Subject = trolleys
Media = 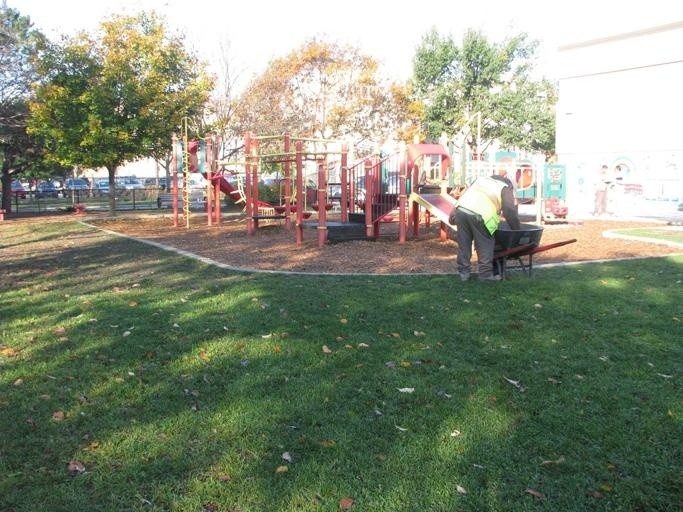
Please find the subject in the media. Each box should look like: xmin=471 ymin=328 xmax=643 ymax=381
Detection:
xmin=493 ymin=220 xmax=579 ymax=282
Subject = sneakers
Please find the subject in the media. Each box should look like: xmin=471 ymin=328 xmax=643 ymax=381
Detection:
xmin=479 ymin=274 xmax=502 ymax=282
xmin=460 ymin=271 xmax=470 ymax=281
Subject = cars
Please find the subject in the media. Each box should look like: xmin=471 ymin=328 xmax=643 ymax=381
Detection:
xmin=0 ymin=174 xmax=201 ymax=208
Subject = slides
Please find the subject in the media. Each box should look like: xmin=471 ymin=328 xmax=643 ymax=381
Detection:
xmin=201 ymin=172 xmax=272 ymax=207
xmin=410 ymin=191 xmax=458 ymax=231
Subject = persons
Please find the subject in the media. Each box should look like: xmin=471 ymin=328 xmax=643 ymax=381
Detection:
xmin=594 ymin=164 xmax=615 ymax=216
xmin=453 ymin=175 xmax=522 ymax=282
xmin=615 ymin=166 xmax=624 ymax=180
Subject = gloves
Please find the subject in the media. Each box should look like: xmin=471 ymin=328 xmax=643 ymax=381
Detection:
xmin=449 ymin=209 xmax=457 ymax=225
